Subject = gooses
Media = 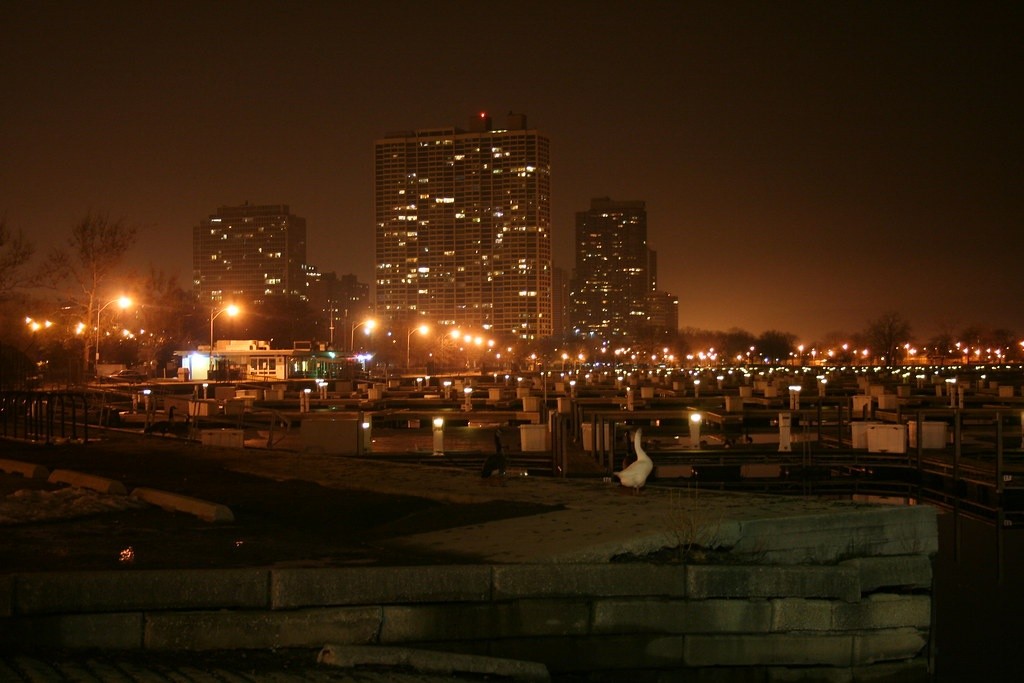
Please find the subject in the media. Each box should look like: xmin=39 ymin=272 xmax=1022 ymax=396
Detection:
xmin=612 ymin=427 xmax=656 ymax=497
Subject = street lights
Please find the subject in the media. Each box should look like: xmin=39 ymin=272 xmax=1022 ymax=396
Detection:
xmin=440 ymin=330 xmax=461 ymax=364
xmin=209 ymin=303 xmax=238 ymax=357
xmin=405 ymin=326 xmax=429 ymax=371
xmin=349 ymin=319 xmax=377 ymax=354
xmin=94 ymin=296 xmax=132 ymax=371
